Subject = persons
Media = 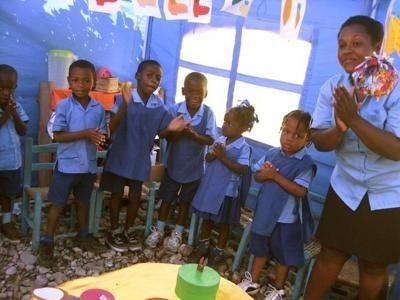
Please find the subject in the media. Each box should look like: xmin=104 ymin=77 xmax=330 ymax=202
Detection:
xmin=190 ymin=99 xmax=258 ymax=264
xmin=144 ymin=70 xmax=217 ymax=254
xmin=235 ymin=110 xmax=317 ymax=300
xmin=0 ymin=63 xmax=29 ymax=244
xmin=39 ymin=59 xmax=109 ymax=260
xmin=98 ymin=60 xmax=187 ymax=253
xmin=302 ymin=14 xmax=400 ymax=300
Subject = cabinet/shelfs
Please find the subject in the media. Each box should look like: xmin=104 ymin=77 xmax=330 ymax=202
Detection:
xmin=38 ymin=81 xmax=165 ymax=213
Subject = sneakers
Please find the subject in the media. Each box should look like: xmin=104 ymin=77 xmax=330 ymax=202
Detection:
xmin=75 ymin=232 xmax=107 ymax=254
xmin=2 ymin=221 xmax=20 ymax=240
xmin=106 ymin=228 xmax=129 ymax=253
xmin=236 ymin=270 xmax=261 ymax=296
xmin=122 ymin=227 xmax=142 ymax=251
xmin=264 ymin=283 xmax=287 ymax=300
xmin=165 ymin=229 xmax=183 ymax=252
xmin=37 ymin=235 xmax=55 ymax=266
xmin=144 ymin=226 xmax=165 ymax=249
xmin=187 ymin=246 xmax=211 ymax=262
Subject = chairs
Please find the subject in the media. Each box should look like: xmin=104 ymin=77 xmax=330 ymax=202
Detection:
xmin=231 ymin=215 xmax=322 ymax=300
xmin=302 ymin=255 xmax=400 ymax=300
xmin=151 ymin=181 xmax=199 ymax=245
xmin=195 ymin=186 xmax=260 ymax=272
xmin=21 ymin=137 xmax=96 ymax=249
xmin=94 ymin=151 xmax=153 ymax=236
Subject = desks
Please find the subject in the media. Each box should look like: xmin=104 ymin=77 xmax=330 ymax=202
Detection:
xmin=31 ymin=263 xmax=255 ymax=300
